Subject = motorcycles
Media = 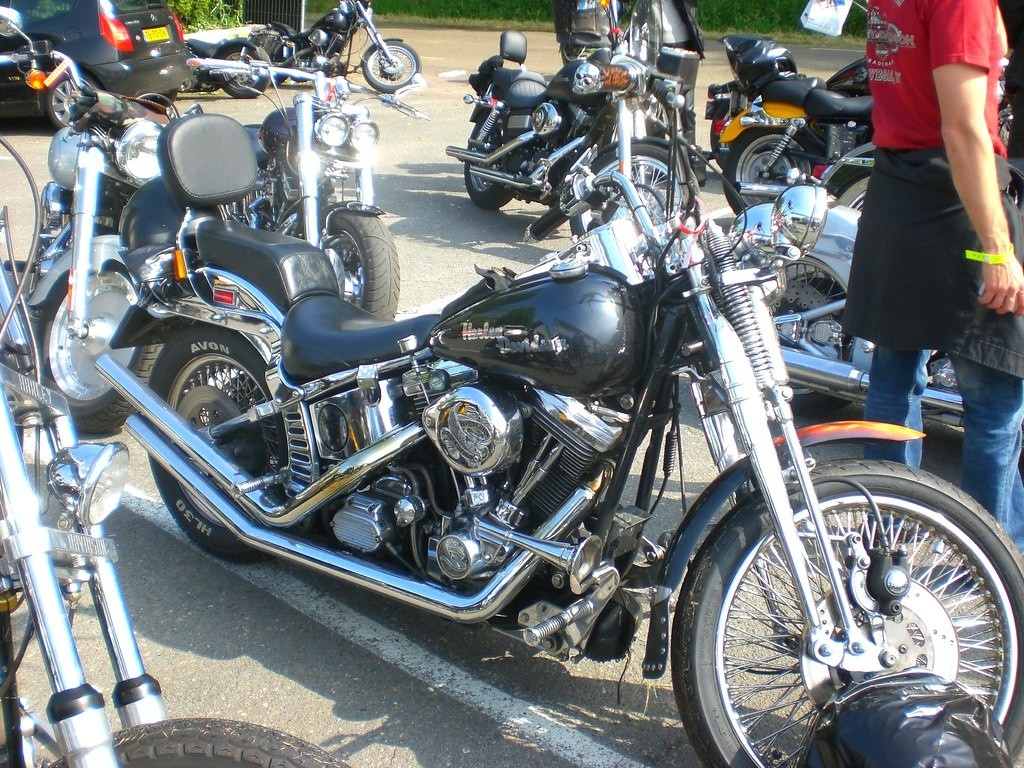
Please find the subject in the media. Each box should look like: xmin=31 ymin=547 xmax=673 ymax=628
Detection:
xmin=440 ymin=0 xmax=1024 ymax=468
xmin=1 ymin=134 xmax=351 ymax=768
xmin=1 ymin=4 xmax=207 ymax=438
xmin=182 ymin=49 xmax=427 ymax=321
xmin=181 ymin=31 xmax=274 ymax=99
xmin=249 ymin=0 xmax=423 ymax=94
xmin=111 ymin=36 xmax=1024 ymax=768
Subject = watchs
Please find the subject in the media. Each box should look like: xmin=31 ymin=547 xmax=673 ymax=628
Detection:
xmin=966 ymin=244 xmax=1015 ymax=264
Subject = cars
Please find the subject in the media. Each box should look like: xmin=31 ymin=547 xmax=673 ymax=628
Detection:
xmin=2 ymin=0 xmax=195 ymax=131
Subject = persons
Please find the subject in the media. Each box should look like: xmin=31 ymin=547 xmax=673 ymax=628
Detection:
xmin=840 ymin=0 xmax=1024 ymax=548
xmin=633 ymin=0 xmax=707 ymax=190
xmin=554 ymin=0 xmax=624 ymax=67
